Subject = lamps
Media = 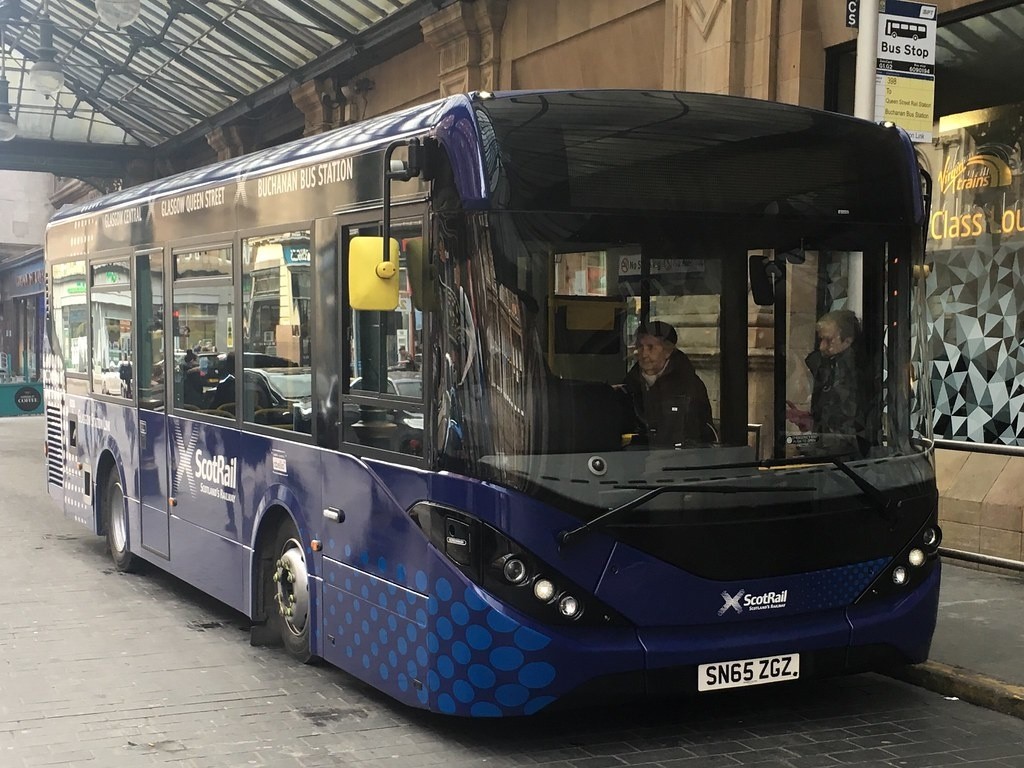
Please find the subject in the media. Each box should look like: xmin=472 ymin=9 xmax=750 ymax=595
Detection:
xmin=0 ymin=0 xmax=141 ymax=140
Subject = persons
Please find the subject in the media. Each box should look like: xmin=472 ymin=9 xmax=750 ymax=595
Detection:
xmin=179 ymin=349 xmax=203 ymax=410
xmin=210 ymin=351 xmax=246 ymax=533
xmin=613 ymin=321 xmax=717 ymax=451
xmin=786 ymin=309 xmax=884 ymax=458
xmin=397 ymin=346 xmax=417 ymax=371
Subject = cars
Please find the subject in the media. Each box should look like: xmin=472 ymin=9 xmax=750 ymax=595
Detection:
xmin=350 ymin=370 xmax=424 ymax=431
xmin=102 ymin=362 xmax=131 ymax=396
xmin=176 ymin=351 xmax=220 ymax=386
xmin=244 ymin=367 xmax=311 ymax=422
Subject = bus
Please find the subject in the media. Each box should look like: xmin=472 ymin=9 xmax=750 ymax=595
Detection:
xmin=43 ymin=87 xmax=943 ymax=724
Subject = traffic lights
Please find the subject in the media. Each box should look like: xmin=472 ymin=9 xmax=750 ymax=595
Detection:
xmin=155 ymin=308 xmax=163 ymax=327
xmin=170 ymin=309 xmax=182 ymax=338
